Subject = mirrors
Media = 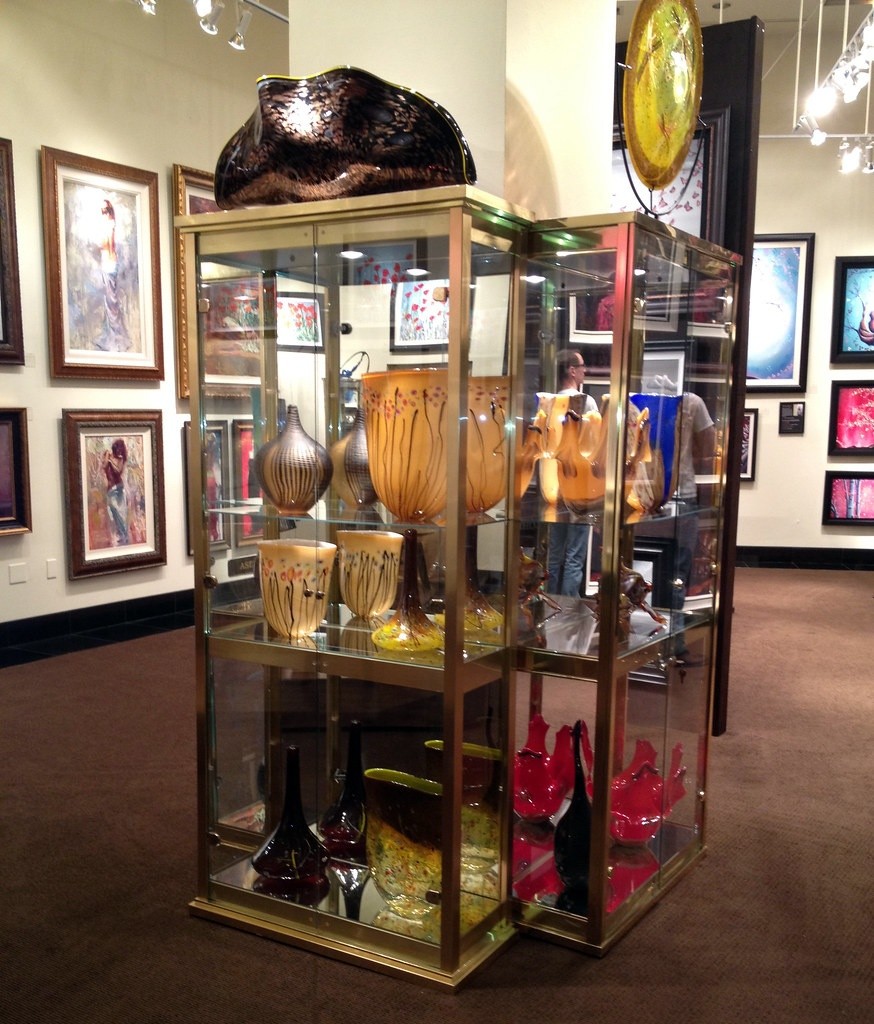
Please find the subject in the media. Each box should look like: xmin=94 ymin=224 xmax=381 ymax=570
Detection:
xmin=265 ymin=253 xmax=508 ymax=904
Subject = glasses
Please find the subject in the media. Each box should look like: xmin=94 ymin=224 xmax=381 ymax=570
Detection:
xmin=573 ymin=364 xmax=586 ymax=368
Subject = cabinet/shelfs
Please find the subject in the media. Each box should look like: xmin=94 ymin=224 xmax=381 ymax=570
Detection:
xmin=175 ymin=178 xmax=735 ymax=985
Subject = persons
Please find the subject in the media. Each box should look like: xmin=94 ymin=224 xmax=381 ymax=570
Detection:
xmin=532 ymin=348 xmax=605 ymax=601
xmin=634 ymin=355 xmax=718 ymax=665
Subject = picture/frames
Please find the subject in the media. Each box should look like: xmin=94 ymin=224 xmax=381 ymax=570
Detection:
xmin=276 ymin=289 xmax=327 ymax=354
xmin=0 ymin=406 xmax=34 ymax=537
xmin=829 ymin=255 xmax=874 ymax=365
xmin=820 ymin=470 xmax=873 ymax=525
xmin=737 ymin=407 xmax=758 ymax=482
xmin=41 ymin=142 xmax=166 ymax=383
xmin=61 ymin=406 xmax=168 ymax=581
xmin=741 ymin=231 xmax=814 ymax=392
xmin=171 ymin=161 xmax=281 ymax=399
xmin=0 ymin=136 xmax=26 ymax=367
xmin=826 ymin=380 xmax=874 ymax=456
xmin=778 ymin=401 xmax=805 ymax=434
xmin=184 ymin=417 xmax=272 ymax=555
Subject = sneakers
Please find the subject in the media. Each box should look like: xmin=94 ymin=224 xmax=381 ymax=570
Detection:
xmin=676 ymin=650 xmax=707 ymax=667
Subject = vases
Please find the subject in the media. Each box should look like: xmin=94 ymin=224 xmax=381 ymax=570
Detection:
xmin=333 ymin=530 xmax=406 ymax=622
xmin=456 ymin=373 xmax=511 ymax=523
xmin=251 ymin=743 xmax=330 ymax=882
xmin=330 ymin=394 xmax=380 ymax=513
xmin=319 ymin=718 xmax=369 ymax=855
xmin=256 ymin=539 xmax=338 ymax=636
xmin=361 ymin=369 xmax=456 ymax=521
xmin=246 ymin=400 xmax=332 ymax=514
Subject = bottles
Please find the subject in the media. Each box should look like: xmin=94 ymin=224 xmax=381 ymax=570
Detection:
xmin=324 ymin=848 xmax=371 ymax=922
xmin=251 ymin=876 xmax=330 ymax=910
xmin=250 ymin=745 xmax=330 ymax=879
xmin=318 ymin=719 xmax=370 ymax=844
xmin=372 ymin=529 xmax=445 ymax=653
xmin=252 ymin=405 xmax=334 ymax=515
xmin=330 ymin=409 xmax=378 ymax=508
xmin=434 ymin=525 xmax=503 ymax=645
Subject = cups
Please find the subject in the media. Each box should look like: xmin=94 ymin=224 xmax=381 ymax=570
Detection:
xmin=256 ymin=539 xmax=338 ymax=638
xmin=336 ymin=529 xmax=405 ymax=618
xmin=600 ymin=392 xmax=685 ymax=514
xmin=363 ymin=368 xmax=451 ymax=521
xmin=465 ymin=375 xmax=509 ymax=514
